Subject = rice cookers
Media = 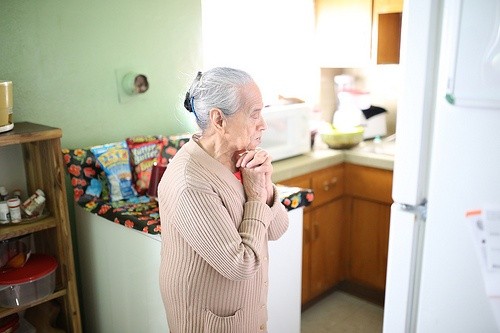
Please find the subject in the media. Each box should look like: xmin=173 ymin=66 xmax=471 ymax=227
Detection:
xmin=0 ymin=81 xmax=14 ymax=133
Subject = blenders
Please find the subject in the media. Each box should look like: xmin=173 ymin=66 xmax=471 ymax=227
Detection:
xmin=333 ymin=73 xmax=370 ymax=129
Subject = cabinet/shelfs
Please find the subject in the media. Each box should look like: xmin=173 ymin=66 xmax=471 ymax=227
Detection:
xmin=273 ymin=164 xmax=393 ymax=311
xmin=311 ymin=0 xmax=404 ymax=71
xmin=0 ymin=121 xmax=83 ymax=333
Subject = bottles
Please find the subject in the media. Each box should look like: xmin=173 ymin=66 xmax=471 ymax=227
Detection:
xmin=0 ymin=185 xmax=45 ymax=223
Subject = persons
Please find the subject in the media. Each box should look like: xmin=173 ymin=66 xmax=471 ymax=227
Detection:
xmin=159 ymin=67 xmax=289 ymax=333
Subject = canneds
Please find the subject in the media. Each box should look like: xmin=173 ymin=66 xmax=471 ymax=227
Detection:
xmin=6 ymin=189 xmax=46 ymax=223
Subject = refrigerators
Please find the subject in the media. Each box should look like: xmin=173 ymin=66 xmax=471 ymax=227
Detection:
xmin=381 ymin=0 xmax=500 ymax=333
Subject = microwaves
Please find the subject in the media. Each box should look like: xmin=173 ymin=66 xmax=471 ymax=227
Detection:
xmin=256 ymin=101 xmax=313 ymax=163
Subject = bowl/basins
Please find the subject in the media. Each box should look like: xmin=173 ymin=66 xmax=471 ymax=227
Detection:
xmin=319 ymin=126 xmax=364 ymax=149
xmin=0 ymin=253 xmax=59 ymax=309
